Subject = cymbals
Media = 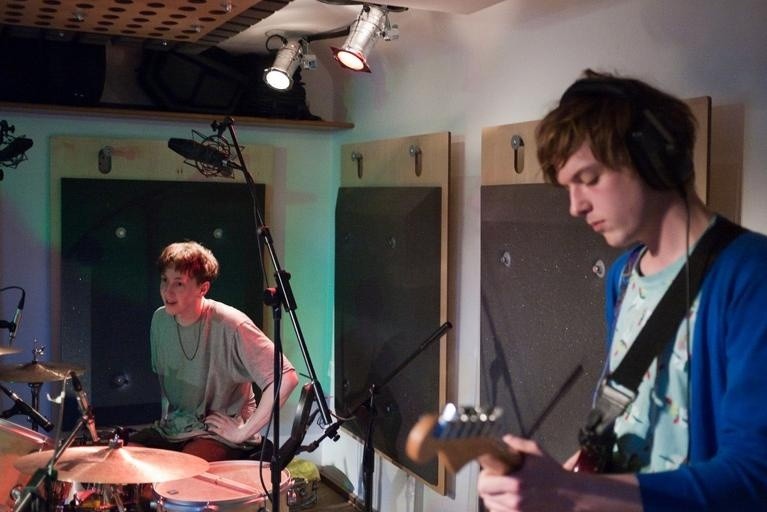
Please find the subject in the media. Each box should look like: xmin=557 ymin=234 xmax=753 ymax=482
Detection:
xmin=14 ymin=446 xmax=209 ymax=485
xmin=0 ymin=363 xmax=83 ymax=382
xmin=0 ymin=347 xmax=23 ymax=355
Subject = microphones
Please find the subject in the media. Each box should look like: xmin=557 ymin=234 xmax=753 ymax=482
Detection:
xmin=7 ymin=291 xmax=25 ymax=346
xmin=168 ymin=138 xmax=239 ymax=173
xmin=0 ymin=136 xmax=33 ymax=163
xmin=70 ymin=369 xmax=98 ymax=445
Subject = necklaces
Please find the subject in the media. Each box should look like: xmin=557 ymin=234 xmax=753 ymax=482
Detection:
xmin=176 ymin=300 xmax=206 ymax=361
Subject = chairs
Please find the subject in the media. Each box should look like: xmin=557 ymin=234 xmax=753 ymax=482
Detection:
xmin=233 ymin=381 xmax=272 ymax=460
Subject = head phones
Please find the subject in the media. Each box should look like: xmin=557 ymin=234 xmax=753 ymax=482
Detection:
xmin=565 ymin=75 xmax=693 ymax=189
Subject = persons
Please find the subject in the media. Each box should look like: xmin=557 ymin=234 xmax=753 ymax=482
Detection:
xmin=475 ymin=70 xmax=767 ymax=512
xmin=123 ymin=240 xmax=299 ymax=510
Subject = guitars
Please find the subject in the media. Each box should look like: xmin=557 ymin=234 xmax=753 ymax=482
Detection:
xmin=407 ymin=404 xmax=644 ymax=476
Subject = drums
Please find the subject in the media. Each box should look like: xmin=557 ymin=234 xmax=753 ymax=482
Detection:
xmin=148 ymin=460 xmax=290 ymax=512
xmin=0 ymin=417 xmax=58 ymax=511
xmin=281 ymin=459 xmax=320 ymax=510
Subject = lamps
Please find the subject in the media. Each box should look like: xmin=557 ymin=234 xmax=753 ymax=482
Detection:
xmin=262 ymin=3 xmax=399 ymax=92
